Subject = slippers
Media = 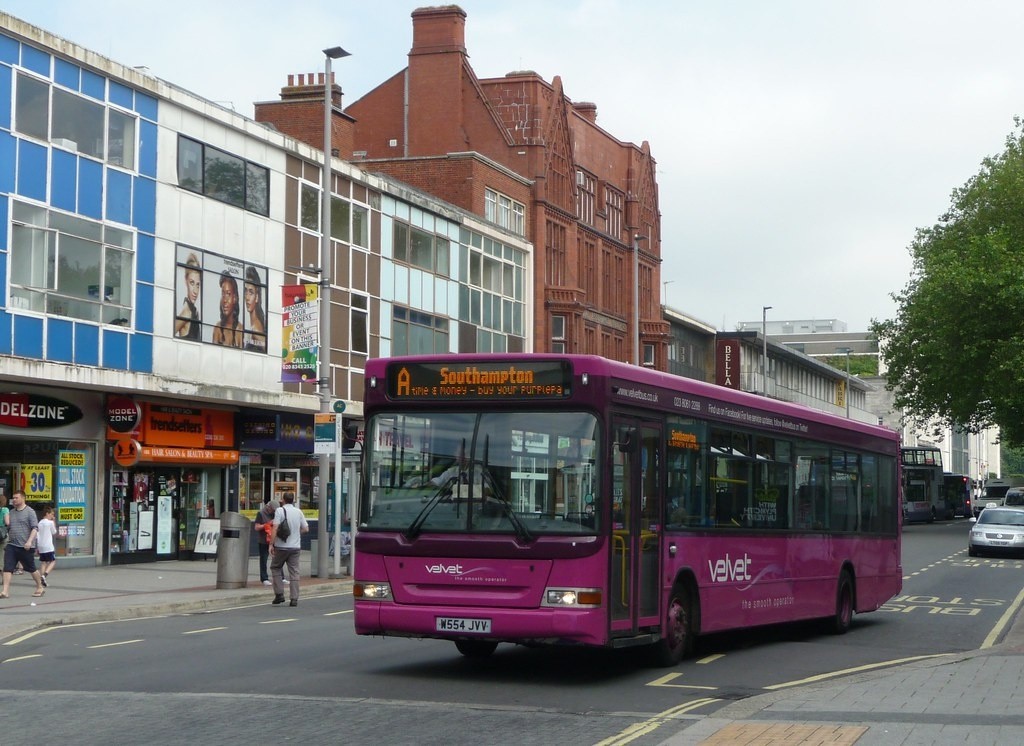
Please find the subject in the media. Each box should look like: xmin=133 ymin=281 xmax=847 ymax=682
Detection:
xmin=32 ymin=589 xmax=45 ymax=597
xmin=0 ymin=592 xmax=9 ymax=598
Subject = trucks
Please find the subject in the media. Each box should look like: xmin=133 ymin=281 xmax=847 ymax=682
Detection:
xmin=973 ymin=479 xmax=1024 ymax=520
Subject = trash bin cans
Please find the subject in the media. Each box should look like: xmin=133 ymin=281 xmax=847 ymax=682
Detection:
xmin=215 ymin=510 xmax=252 ymax=589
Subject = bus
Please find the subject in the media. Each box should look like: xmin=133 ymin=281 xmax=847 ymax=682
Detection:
xmin=352 ymin=352 xmax=904 ymax=667
xmin=901 ymin=446 xmax=975 ymax=524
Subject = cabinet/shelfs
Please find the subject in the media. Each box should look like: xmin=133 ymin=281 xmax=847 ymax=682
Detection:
xmin=111 ymin=471 xmax=124 ymax=552
xmin=180 ymin=481 xmax=202 ymax=549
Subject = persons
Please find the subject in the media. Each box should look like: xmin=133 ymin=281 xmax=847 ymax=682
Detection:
xmin=254 ymin=500 xmax=291 ymax=587
xmin=37 ymin=506 xmax=58 ymax=588
xmin=242 ymin=267 xmax=266 ymax=351
xmin=0 ymin=495 xmax=10 ymax=571
xmin=267 ymin=492 xmax=309 ymax=607
xmin=175 ymin=253 xmax=201 ymax=341
xmin=213 ymin=269 xmax=242 ymax=349
xmin=330 ymin=531 xmax=360 ymax=576
xmin=411 ymin=440 xmax=492 ymax=497
xmin=0 ymin=490 xmax=45 ymax=598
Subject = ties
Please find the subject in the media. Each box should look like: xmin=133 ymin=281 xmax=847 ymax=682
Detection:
xmin=461 ymin=472 xmax=468 ymax=484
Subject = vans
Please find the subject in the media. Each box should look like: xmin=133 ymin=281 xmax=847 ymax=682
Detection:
xmin=1001 ymin=487 xmax=1024 ymax=506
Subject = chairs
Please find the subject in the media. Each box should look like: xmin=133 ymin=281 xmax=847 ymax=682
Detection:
xmin=639 ymin=485 xmax=887 ymax=531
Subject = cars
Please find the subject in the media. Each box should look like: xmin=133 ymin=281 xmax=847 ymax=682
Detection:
xmin=968 ymin=506 xmax=1024 ymax=557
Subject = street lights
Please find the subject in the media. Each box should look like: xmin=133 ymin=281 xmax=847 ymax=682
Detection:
xmin=318 ymin=46 xmax=351 ymax=580
xmin=762 ymin=307 xmax=772 ymax=397
xmin=846 ymin=350 xmax=854 ymax=417
xmin=633 ymin=234 xmax=648 ymax=365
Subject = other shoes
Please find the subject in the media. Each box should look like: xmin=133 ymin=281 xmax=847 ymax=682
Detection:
xmin=289 ymin=598 xmax=298 ymax=606
xmin=263 ymin=580 xmax=273 ymax=586
xmin=41 ymin=574 xmax=48 ymax=586
xmin=271 ymin=593 xmax=285 ymax=604
xmin=282 ymin=579 xmax=291 ymax=584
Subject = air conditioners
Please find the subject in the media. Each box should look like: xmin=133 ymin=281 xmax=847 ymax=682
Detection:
xmin=576 ymin=171 xmax=585 ymax=186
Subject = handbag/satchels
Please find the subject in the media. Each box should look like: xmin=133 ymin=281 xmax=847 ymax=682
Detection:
xmin=276 ymin=519 xmax=290 ymax=543
xmin=264 ymin=520 xmax=272 ymax=543
xmin=0 ymin=526 xmax=7 ymax=539
